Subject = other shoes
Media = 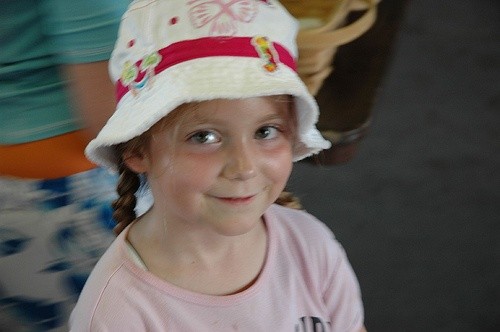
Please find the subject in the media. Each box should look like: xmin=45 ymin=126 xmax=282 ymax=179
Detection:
xmin=320 ymin=117 xmax=373 ymax=148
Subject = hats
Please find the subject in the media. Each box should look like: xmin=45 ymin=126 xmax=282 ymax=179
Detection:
xmin=85 ymin=0 xmax=332 ymax=175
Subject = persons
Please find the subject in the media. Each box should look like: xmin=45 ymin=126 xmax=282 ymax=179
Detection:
xmin=68 ymin=1 xmax=367 ymax=332
xmin=2 ymin=1 xmax=156 ymax=332
